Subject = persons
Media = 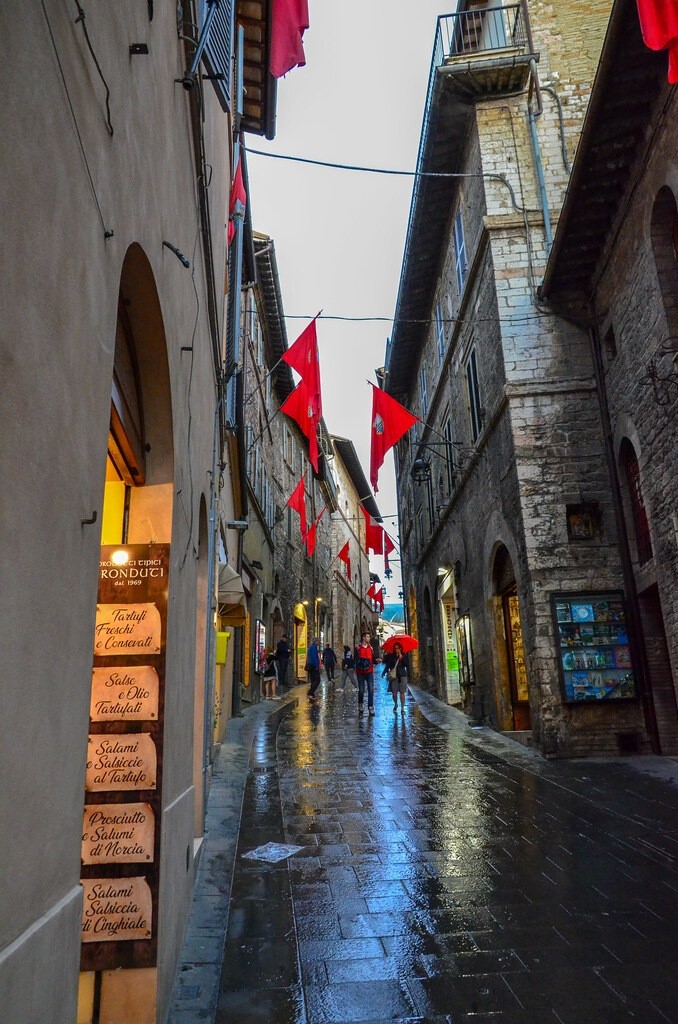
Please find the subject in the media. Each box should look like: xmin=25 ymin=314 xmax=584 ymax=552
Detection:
xmin=323 ymin=643 xmax=338 ymax=682
xmin=263 ymin=644 xmax=282 ymax=700
xmin=382 ymin=642 xmax=408 ymax=712
xmin=306 ymin=637 xmax=321 ymax=703
xmin=335 ymin=646 xmax=359 ymax=693
xmin=275 ymin=633 xmax=292 ymax=687
xmin=355 ymin=631 xmax=382 ymax=714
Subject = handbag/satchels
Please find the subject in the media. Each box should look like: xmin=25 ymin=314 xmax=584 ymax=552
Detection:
xmin=353 ymin=657 xmax=371 ymax=670
xmin=304 ymin=664 xmax=314 ymax=671
xmin=387 ymin=668 xmax=396 ymax=681
xmin=342 ymin=659 xmax=345 ymax=670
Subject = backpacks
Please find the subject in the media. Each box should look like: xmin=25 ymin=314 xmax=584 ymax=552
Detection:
xmin=259 ymin=658 xmax=273 ymax=674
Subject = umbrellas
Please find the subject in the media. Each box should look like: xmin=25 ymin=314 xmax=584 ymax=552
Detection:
xmin=381 ymin=635 xmax=419 ymax=653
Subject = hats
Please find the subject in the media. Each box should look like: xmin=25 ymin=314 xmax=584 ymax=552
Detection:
xmin=312 ymin=638 xmax=319 ymax=642
xmin=280 ymin=633 xmax=288 ymax=638
xmin=343 ymin=645 xmax=349 ymax=648
xmin=325 ymin=643 xmax=330 ymax=644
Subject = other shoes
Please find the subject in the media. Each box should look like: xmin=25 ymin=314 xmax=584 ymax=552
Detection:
xmin=331 ymin=679 xmax=336 ymax=684
xmin=358 ymin=704 xmax=365 ymax=711
xmin=266 ymin=695 xmax=271 ymax=699
xmin=309 ymin=694 xmax=317 ymax=700
xmin=336 ymin=688 xmax=344 ymax=692
xmin=368 ymin=706 xmax=375 ymax=714
xmin=328 ymin=681 xmax=331 ymax=684
xmin=393 ymin=705 xmax=398 ymax=712
xmin=307 ymin=693 xmax=309 ymax=697
xmin=272 ymin=695 xmax=281 ymax=699
xmin=349 ymin=687 xmax=359 ymax=691
xmin=401 ymin=707 xmax=404 ymax=712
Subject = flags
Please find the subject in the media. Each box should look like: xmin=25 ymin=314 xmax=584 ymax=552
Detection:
xmin=269 ymin=0 xmax=310 ymax=80
xmin=282 ymin=320 xmax=420 ymax=611
